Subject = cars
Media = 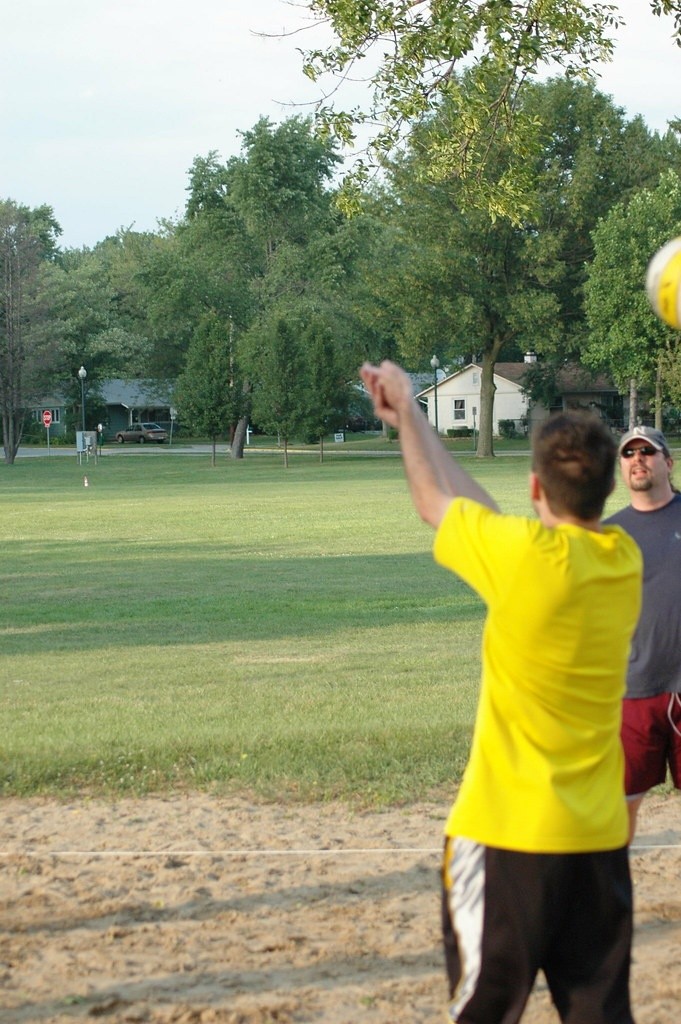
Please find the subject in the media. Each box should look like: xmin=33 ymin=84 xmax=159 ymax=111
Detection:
xmin=116 ymin=423 xmax=168 ymax=444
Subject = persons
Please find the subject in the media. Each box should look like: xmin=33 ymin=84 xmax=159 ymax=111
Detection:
xmin=601 ymin=427 xmax=681 ymax=848
xmin=359 ymin=358 xmax=646 ymax=1024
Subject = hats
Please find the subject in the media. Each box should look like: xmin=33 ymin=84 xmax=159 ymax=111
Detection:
xmin=618 ymin=426 xmax=671 ymax=459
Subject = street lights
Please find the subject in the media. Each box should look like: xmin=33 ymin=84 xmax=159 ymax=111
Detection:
xmin=430 ymin=355 xmax=439 ymax=434
xmin=78 ymin=365 xmax=87 ymax=431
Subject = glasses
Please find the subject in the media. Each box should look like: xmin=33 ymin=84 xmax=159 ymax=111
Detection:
xmin=621 ymin=445 xmax=665 ymax=460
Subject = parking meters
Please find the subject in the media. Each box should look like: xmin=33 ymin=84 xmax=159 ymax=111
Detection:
xmin=98 ymin=424 xmax=104 ymax=456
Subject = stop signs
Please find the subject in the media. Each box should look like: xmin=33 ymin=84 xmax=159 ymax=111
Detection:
xmin=43 ymin=411 xmax=52 ymax=425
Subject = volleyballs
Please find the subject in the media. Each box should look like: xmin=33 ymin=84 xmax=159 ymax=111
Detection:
xmin=643 ymin=232 xmax=681 ymax=334
